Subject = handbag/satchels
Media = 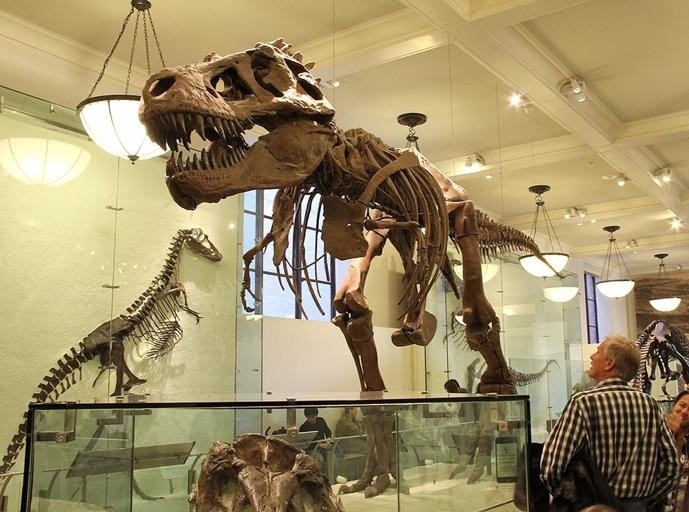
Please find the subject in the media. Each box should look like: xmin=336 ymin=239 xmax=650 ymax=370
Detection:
xmin=548 ymin=448 xmax=625 ymax=512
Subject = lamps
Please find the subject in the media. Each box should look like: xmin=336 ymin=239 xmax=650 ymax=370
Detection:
xmin=543 ymin=276 xmax=579 ymax=303
xmin=617 ymin=176 xmax=625 ymax=186
xmin=661 ymin=166 xmax=673 ymax=181
xmin=595 ymin=225 xmax=636 ymax=299
xmin=76 ymin=0 xmax=180 ymax=165
xmin=648 ymin=254 xmax=682 ymax=312
xmin=455 ymin=263 xmax=498 ymax=282
xmin=330 ymin=80 xmax=340 ymax=87
xmin=571 ymin=78 xmax=586 ymax=102
xmin=564 ymin=206 xmax=585 ymax=219
xmin=518 ymin=185 xmax=569 ymax=279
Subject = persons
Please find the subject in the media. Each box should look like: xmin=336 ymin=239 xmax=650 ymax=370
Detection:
xmin=335 ymin=407 xmax=368 ymax=454
xmin=444 ymin=379 xmax=461 ymax=423
xmin=539 ymin=334 xmax=689 ymax=511
xmin=299 ymin=408 xmax=333 ymax=457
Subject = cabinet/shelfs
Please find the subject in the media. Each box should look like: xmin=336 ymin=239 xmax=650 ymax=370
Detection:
xmin=20 ymin=395 xmax=534 ymax=512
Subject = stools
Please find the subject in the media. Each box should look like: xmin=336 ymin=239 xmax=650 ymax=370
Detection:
xmin=343 ymin=451 xmax=365 ymax=480
